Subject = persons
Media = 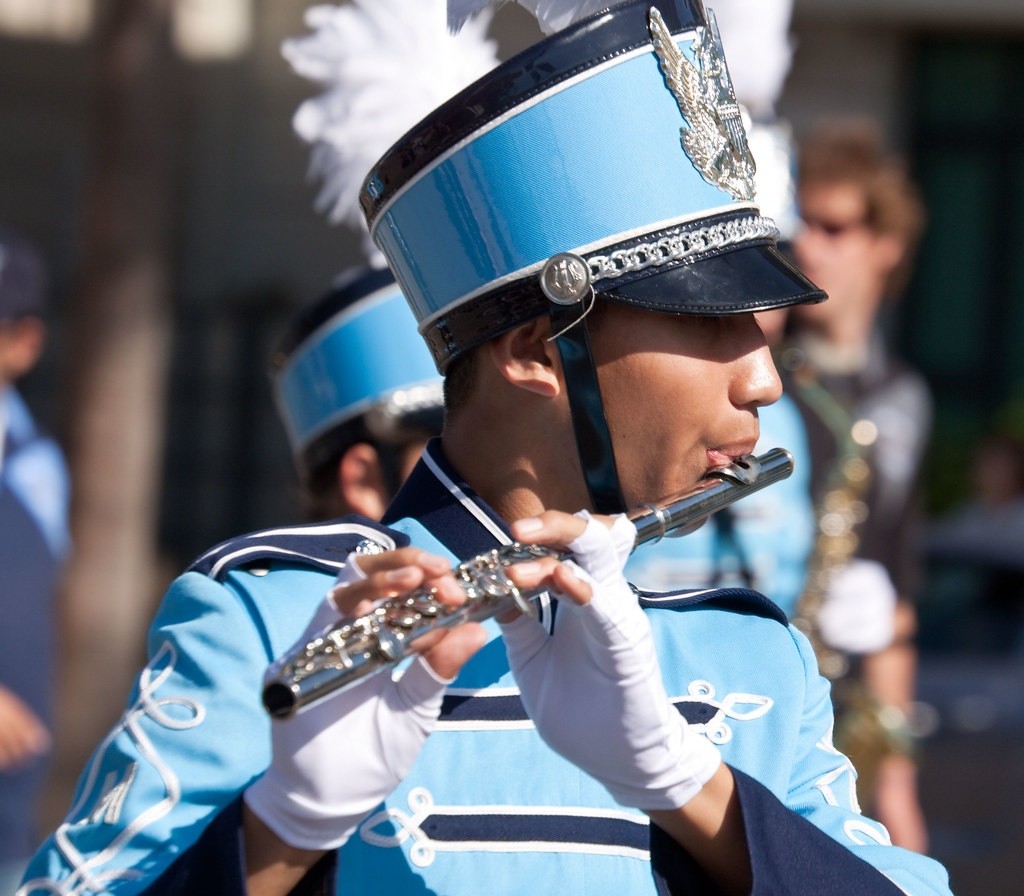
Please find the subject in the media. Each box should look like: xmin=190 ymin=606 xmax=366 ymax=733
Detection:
xmin=0 ymin=2 xmax=1024 ymax=896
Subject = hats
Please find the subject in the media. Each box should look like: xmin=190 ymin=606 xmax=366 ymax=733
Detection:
xmin=261 ymin=267 xmax=454 ymax=485
xmin=358 ymin=0 xmax=833 ymax=378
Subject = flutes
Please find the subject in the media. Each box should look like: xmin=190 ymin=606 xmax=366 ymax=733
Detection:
xmin=258 ymin=447 xmax=796 ymax=722
